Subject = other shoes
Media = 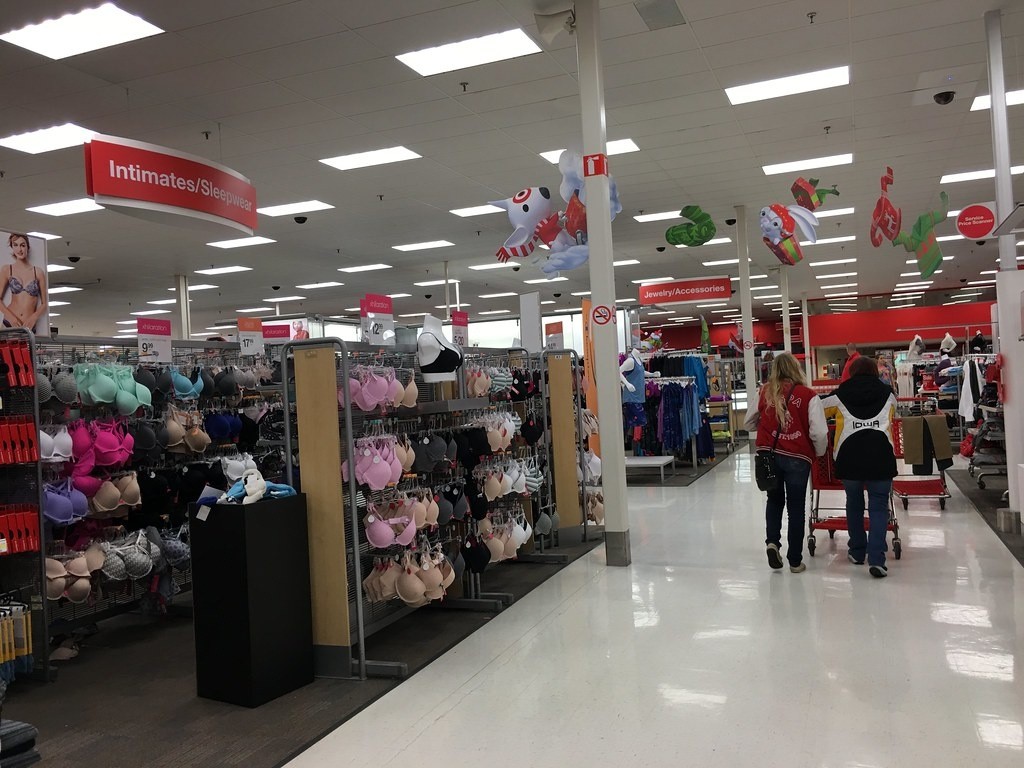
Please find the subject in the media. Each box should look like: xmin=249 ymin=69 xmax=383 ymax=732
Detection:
xmin=848 ymin=554 xmax=864 ymax=564
xmin=869 ymin=565 xmax=887 ymax=577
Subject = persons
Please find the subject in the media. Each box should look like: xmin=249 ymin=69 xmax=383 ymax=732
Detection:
xmin=940 ymin=332 xmax=957 ymax=357
xmin=0 ymin=234 xmax=48 ymax=334
xmin=912 ymin=334 xmax=922 ymax=354
xmin=819 ymin=357 xmax=899 ymax=576
xmin=418 ymin=315 xmax=463 ymax=382
xmin=876 ymin=354 xmax=897 ymax=386
xmin=292 ymin=321 xmax=309 ymax=339
xmin=840 ymin=342 xmax=861 ymax=382
xmin=744 ymin=352 xmax=829 ymax=573
xmin=620 ymin=348 xmax=660 ymax=456
xmin=971 ymin=329 xmax=987 ymax=354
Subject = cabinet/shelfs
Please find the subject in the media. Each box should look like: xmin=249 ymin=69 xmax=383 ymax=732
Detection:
xmin=704 ymin=364 xmax=735 ymax=454
xmin=279 ymin=337 xmax=542 ymax=682
xmin=535 ymin=348 xmax=593 ymax=546
xmin=918 ymin=369 xmax=1008 ymax=488
xmin=0 ymin=327 xmax=299 ymax=688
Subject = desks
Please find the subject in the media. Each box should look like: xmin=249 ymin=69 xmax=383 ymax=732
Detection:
xmin=625 ymin=455 xmax=677 ymax=485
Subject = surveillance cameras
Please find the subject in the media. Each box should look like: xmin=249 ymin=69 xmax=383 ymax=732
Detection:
xmin=960 ymin=279 xmax=967 ymax=282
xmin=933 ymin=91 xmax=956 ymax=105
xmin=944 ymin=294 xmax=949 ymax=296
xmin=553 ymin=293 xmax=561 ymax=297
xmin=272 ymin=286 xmax=280 ymax=290
xmin=731 ymin=290 xmax=736 ymax=294
xmin=293 ymin=217 xmax=307 ymax=224
xmin=68 ymin=257 xmax=80 ymax=262
xmin=513 ymin=267 xmax=519 ymax=271
xmin=975 ymin=241 xmax=986 ymax=246
xmin=425 ymin=295 xmax=432 ymax=298
xmin=725 ymin=219 xmax=736 ymax=225
xmin=656 ymin=247 xmax=665 ymax=252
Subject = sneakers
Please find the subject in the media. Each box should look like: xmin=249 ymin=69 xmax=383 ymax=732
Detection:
xmin=767 ymin=543 xmax=783 ymax=569
xmin=790 ymin=562 xmax=806 ymax=573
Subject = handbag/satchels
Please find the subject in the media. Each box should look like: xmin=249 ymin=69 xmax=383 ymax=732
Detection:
xmin=754 ymin=456 xmax=781 ymax=491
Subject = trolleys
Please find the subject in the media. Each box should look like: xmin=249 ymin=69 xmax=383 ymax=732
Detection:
xmin=891 ymin=397 xmax=953 ymax=510
xmin=804 ymin=416 xmax=903 ymax=561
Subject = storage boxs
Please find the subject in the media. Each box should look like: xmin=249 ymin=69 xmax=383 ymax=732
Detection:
xmin=1 ymin=340 xmax=40 ymax=684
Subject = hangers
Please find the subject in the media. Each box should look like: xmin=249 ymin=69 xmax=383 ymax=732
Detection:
xmin=645 ymin=347 xmax=702 ymax=387
xmin=898 ymin=353 xmax=996 ymax=367
xmin=37 ymin=345 xmax=299 ymax=565
xmin=345 ymin=351 xmax=605 ymax=571
